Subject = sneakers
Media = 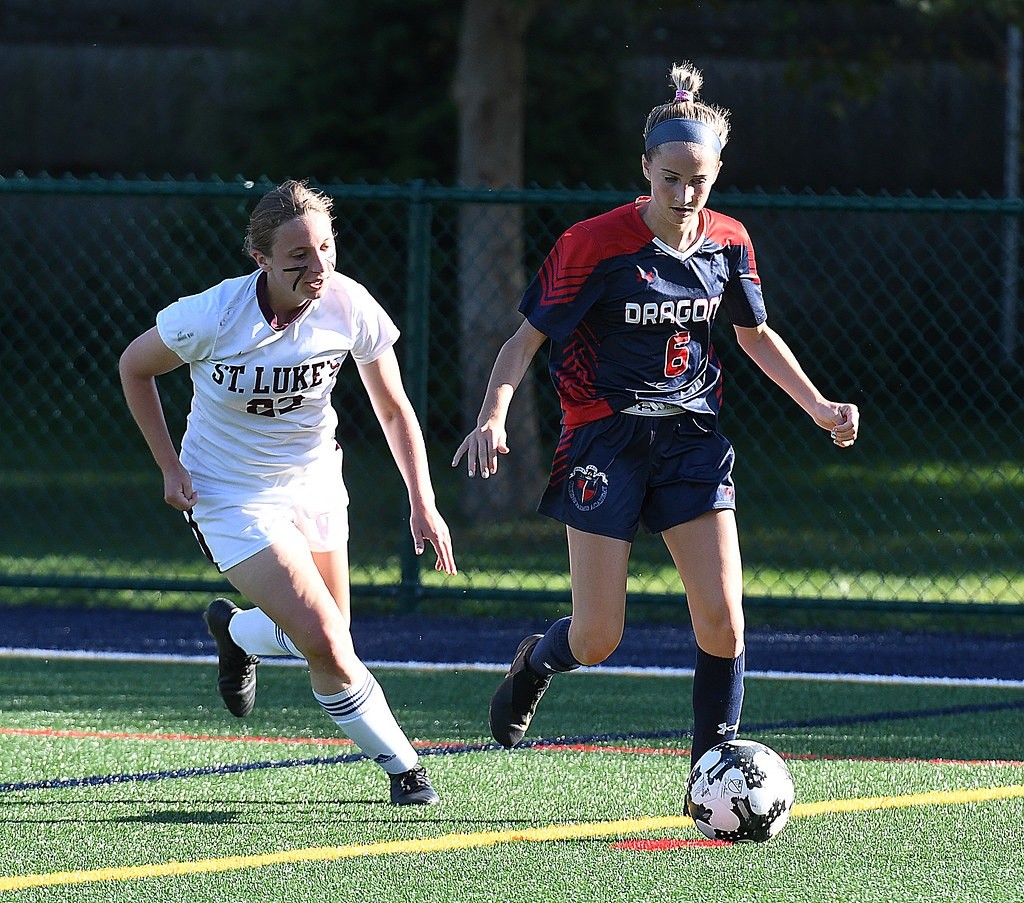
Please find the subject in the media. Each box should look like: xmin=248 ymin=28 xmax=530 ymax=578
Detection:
xmin=388 ymin=758 xmax=439 ymax=806
xmin=203 ymin=596 xmax=260 ymax=717
xmin=489 ymin=634 xmax=553 ymax=750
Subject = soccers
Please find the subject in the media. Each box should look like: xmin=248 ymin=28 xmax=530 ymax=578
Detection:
xmin=685 ymin=739 xmax=796 ymax=843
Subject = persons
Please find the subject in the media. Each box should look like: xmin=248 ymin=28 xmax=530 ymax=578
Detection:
xmin=119 ymin=180 xmax=459 ymax=805
xmin=452 ymin=68 xmax=860 ymax=814
